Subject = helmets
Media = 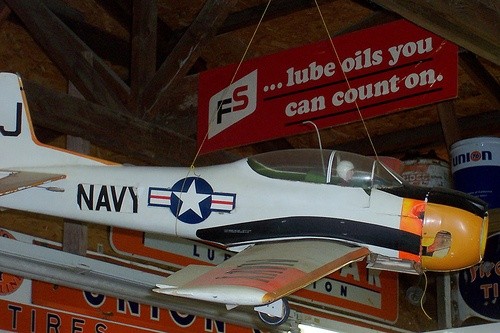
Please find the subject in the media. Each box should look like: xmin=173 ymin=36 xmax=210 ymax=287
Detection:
xmin=336 ymin=160 xmax=355 ymax=181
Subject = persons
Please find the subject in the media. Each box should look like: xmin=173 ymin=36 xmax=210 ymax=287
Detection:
xmin=337 ymin=161 xmax=354 ymax=184
